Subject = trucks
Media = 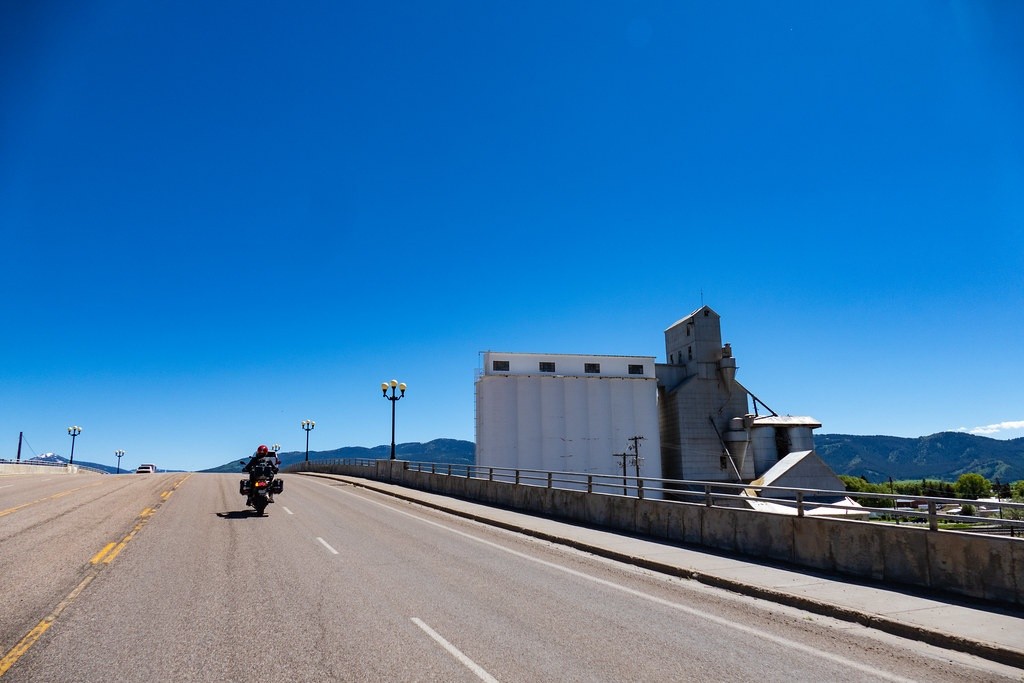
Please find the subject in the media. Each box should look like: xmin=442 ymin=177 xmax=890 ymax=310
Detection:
xmin=137 ymin=463 xmax=154 ymax=473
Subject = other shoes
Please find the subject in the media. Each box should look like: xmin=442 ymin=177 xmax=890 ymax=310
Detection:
xmin=246 ymin=500 xmax=251 ymax=505
xmin=269 ymin=496 xmax=274 ymax=502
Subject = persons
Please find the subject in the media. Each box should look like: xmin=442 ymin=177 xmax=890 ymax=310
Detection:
xmin=242 ymin=445 xmax=274 ymax=506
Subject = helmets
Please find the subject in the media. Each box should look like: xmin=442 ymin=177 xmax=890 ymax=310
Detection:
xmin=258 ymin=445 xmax=269 ymax=455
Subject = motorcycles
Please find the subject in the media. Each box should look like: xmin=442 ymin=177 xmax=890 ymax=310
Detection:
xmin=239 ymin=459 xmax=284 ymax=517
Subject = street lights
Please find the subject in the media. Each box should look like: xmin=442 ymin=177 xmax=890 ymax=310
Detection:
xmin=115 ymin=450 xmax=124 ymax=474
xmin=68 ymin=426 xmax=82 ymax=465
xmin=271 ymin=444 xmax=280 ymax=459
xmin=302 ymin=420 xmax=315 ymax=461
xmin=381 ymin=380 xmax=407 ymax=460
